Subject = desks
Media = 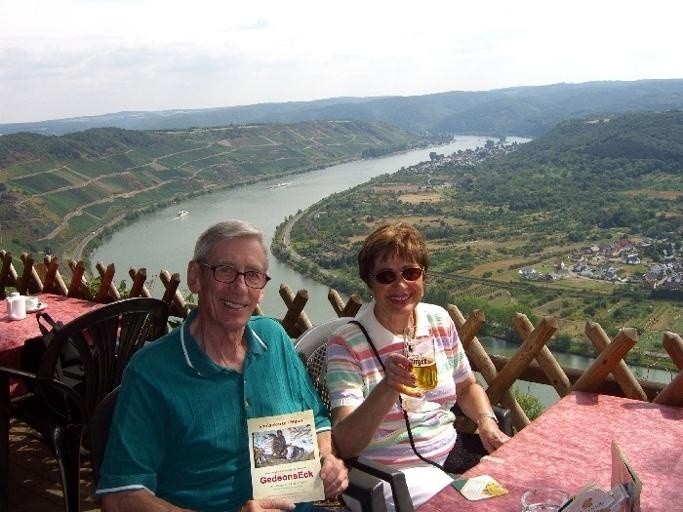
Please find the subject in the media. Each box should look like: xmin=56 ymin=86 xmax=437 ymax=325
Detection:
xmin=411 ymin=391 xmax=683 ymax=512
xmin=0 ymin=290 xmax=111 ymax=454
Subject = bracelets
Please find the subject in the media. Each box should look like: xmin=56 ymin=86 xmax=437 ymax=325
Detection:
xmin=474 ymin=413 xmax=499 ymax=423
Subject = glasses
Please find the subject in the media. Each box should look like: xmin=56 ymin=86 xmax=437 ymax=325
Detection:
xmin=196 ymin=259 xmax=271 ymax=289
xmin=367 ymin=266 xmax=425 ymax=284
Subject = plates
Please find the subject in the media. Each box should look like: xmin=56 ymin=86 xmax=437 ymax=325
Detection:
xmin=27 ymin=303 xmax=48 ymax=313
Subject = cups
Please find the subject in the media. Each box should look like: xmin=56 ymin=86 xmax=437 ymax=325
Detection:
xmin=403 ymin=326 xmax=438 ymax=393
xmin=520 ymin=488 xmax=570 ymax=512
xmin=25 ymin=297 xmax=38 ymax=309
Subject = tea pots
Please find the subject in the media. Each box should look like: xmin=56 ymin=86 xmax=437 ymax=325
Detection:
xmin=6 ymin=292 xmax=26 ymax=321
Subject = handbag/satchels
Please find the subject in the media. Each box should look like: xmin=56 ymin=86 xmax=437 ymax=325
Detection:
xmin=443 ymin=427 xmax=491 ymax=473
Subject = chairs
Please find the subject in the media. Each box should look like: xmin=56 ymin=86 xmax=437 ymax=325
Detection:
xmin=292 ymin=314 xmax=513 ymax=512
xmin=32 ymin=296 xmax=168 ymax=512
xmin=88 ymin=386 xmax=390 ymax=512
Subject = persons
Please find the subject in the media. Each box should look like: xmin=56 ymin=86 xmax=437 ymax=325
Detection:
xmin=95 ymin=220 xmax=350 ymax=511
xmin=325 ymin=222 xmax=511 ymax=511
